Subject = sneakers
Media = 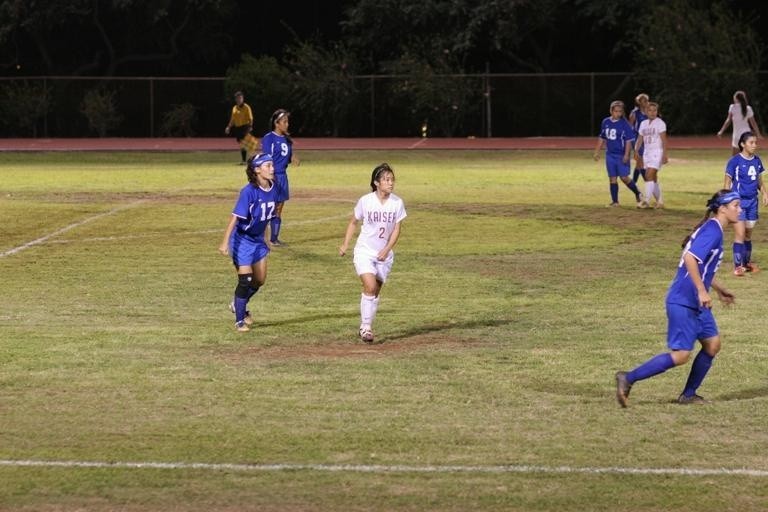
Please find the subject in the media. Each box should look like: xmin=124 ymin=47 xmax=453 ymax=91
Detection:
xmin=637 ymin=192 xmax=643 ymax=202
xmin=236 ymin=321 xmax=249 ymax=331
xmin=605 ymin=202 xmax=620 ymax=208
xmin=678 ymin=393 xmax=711 ymax=404
xmin=637 ymin=200 xmax=649 ymax=209
xmin=734 ymin=266 xmax=745 ymax=276
xmin=616 ymin=371 xmax=632 ymax=407
xmin=361 ymin=330 xmax=374 ymax=341
xmin=743 ymin=263 xmax=759 ymax=272
xmin=653 ymin=203 xmax=663 ymax=209
xmin=243 ymin=316 xmax=253 ymax=325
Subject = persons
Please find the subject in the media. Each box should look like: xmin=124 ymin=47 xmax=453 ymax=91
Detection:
xmin=615 ymin=189 xmax=743 ymax=409
xmin=262 ymin=109 xmax=300 ymax=246
xmin=717 ymin=91 xmax=764 ymax=156
xmin=340 ymin=163 xmax=407 ymax=341
xmin=724 ymin=132 xmax=768 ymax=276
xmin=592 ymin=101 xmax=643 ymax=209
xmin=219 ymin=151 xmax=280 ymax=332
xmin=634 ymin=102 xmax=668 ymax=209
xmin=225 ymin=92 xmax=254 ymax=164
xmin=628 ymin=94 xmax=650 ymax=184
